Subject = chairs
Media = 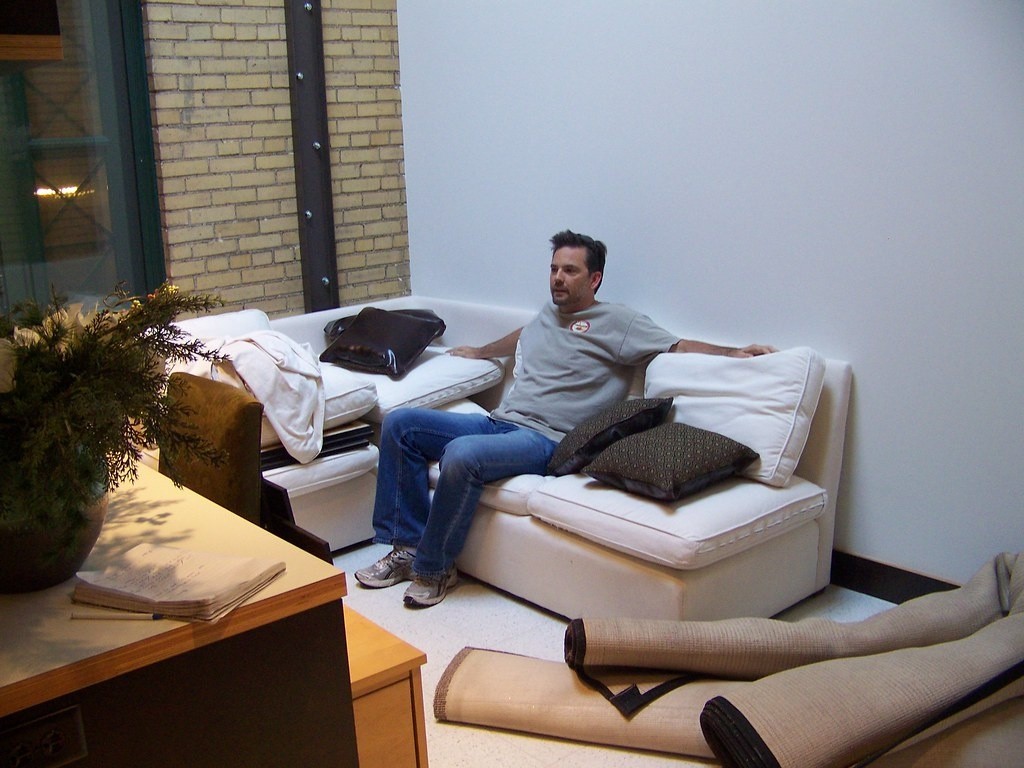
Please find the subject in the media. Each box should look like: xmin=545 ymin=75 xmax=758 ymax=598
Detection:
xmin=159 ymin=372 xmax=264 ymax=528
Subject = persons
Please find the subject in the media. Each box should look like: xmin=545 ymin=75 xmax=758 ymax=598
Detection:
xmin=354 ymin=229 xmax=777 ymax=608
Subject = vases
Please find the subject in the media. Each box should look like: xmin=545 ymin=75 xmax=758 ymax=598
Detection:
xmin=0 ymin=459 xmax=110 ymax=596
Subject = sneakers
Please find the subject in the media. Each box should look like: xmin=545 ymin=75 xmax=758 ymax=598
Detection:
xmin=404 ymin=560 xmax=459 ymax=607
xmin=354 ymin=548 xmax=417 ymax=588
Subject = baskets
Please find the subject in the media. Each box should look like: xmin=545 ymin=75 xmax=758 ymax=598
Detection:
xmin=0 ymin=492 xmax=107 ymax=593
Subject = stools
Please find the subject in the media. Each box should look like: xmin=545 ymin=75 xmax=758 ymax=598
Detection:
xmin=342 ymin=603 xmax=429 ymax=768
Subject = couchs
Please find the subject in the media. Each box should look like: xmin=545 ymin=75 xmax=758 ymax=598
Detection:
xmin=260 ymin=294 xmax=851 ymax=622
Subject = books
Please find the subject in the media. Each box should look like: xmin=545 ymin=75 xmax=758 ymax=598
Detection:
xmin=68 ymin=542 xmax=289 ymax=624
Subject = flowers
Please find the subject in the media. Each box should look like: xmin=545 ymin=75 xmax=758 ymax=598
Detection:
xmin=0 ymin=274 xmax=230 ymax=562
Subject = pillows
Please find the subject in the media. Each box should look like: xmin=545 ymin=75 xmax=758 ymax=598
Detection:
xmin=319 ymin=306 xmax=442 ymax=379
xmin=136 ymin=309 xmax=271 ymax=422
xmin=315 ymin=345 xmax=505 ymax=421
xmin=261 ymin=361 xmax=379 ymax=448
xmin=323 ymin=308 xmax=446 ymax=342
xmin=580 ymin=422 xmax=761 ymax=502
xmin=644 ymin=346 xmax=828 ymax=487
xmin=545 ymin=396 xmax=675 ymax=476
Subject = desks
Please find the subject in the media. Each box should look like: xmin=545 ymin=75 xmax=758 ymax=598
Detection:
xmin=0 ymin=457 xmax=360 ymax=768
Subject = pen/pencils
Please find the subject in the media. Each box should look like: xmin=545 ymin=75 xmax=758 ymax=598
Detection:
xmin=72 ymin=612 xmax=163 ymax=621
